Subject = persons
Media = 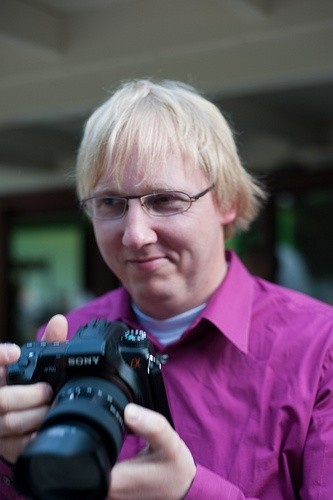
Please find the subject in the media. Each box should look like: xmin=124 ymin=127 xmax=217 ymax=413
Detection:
xmin=0 ymin=79 xmax=333 ymax=499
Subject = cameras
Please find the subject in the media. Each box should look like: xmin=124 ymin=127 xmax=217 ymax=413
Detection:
xmin=5 ymin=317 xmax=166 ymax=500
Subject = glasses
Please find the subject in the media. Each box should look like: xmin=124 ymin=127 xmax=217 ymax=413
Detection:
xmin=79 ymin=183 xmax=216 ymax=220
